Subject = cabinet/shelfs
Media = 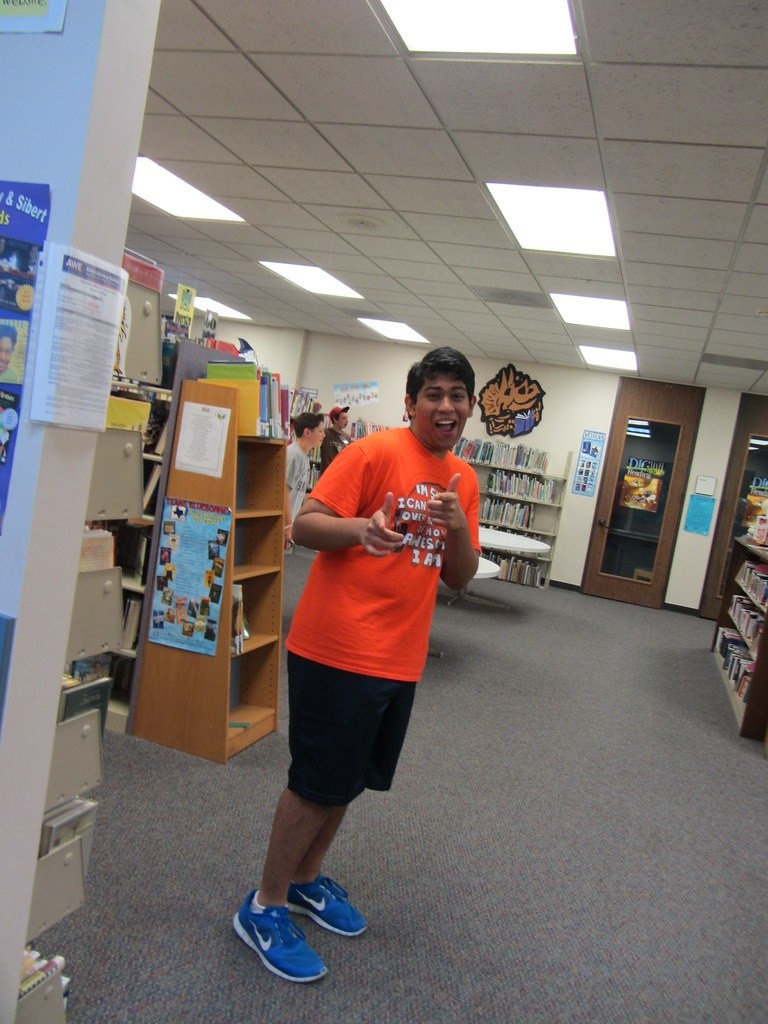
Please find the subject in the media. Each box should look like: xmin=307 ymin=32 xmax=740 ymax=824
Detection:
xmin=710 ymin=536 xmax=768 ymax=741
xmin=11 ymin=331 xmax=282 ymax=1024
xmin=309 ymin=440 xmax=572 ymax=590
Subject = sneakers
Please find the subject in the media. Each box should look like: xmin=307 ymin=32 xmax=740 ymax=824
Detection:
xmin=232 ymin=889 xmax=329 ymax=982
xmin=286 ymin=877 xmax=368 ymax=937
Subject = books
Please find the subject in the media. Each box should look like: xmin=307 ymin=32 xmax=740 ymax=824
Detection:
xmin=483 ymin=498 xmax=536 ymax=529
xmin=486 ymin=470 xmax=557 ymax=503
xmin=19 ymin=248 xmax=188 ymax=1024
xmin=482 ymin=551 xmax=543 ymax=587
xmin=351 ymin=419 xmax=390 ymax=442
xmin=207 ymin=360 xmax=334 ymax=490
xmin=716 ymin=496 xmax=768 ymax=704
xmin=453 ymin=437 xmax=549 ymax=473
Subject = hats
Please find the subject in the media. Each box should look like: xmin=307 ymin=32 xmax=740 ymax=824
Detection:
xmin=330 ymin=407 xmax=350 ymax=421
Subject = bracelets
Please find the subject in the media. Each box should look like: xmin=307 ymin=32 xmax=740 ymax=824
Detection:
xmin=284 ymin=525 xmax=293 ymax=530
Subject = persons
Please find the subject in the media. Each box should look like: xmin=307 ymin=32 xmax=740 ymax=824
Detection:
xmin=318 ymin=406 xmax=350 ymax=481
xmin=232 ymin=347 xmax=483 ymax=987
xmin=0 ymin=325 xmax=17 ymax=383
xmin=284 ymin=412 xmax=326 ymax=548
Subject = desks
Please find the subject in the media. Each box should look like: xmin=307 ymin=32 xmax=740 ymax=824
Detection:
xmin=444 ymin=527 xmax=550 ymax=613
